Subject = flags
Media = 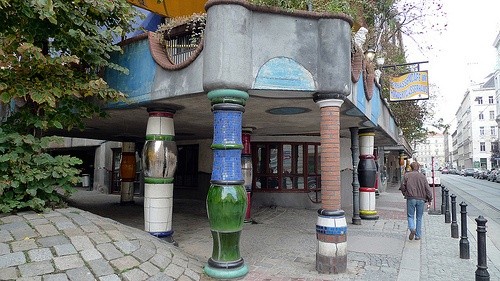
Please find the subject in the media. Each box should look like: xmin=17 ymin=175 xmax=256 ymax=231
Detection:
xmin=391 ymin=72 xmax=428 ymax=99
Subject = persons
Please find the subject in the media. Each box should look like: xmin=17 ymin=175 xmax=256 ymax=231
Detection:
xmin=404 ymin=161 xmax=411 ymax=173
xmin=398 ymin=162 xmax=432 ymax=239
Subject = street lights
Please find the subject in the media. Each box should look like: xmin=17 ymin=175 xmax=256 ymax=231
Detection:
xmin=430 ymin=150 xmax=437 ymax=211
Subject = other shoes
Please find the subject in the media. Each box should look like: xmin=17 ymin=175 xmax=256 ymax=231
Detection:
xmin=415 ymin=237 xmax=420 ymax=240
xmin=409 ymin=230 xmax=415 ymax=240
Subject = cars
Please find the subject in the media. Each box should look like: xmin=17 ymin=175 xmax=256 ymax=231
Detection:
xmin=420 ymin=168 xmax=441 ymax=187
xmin=442 ymin=168 xmax=500 ymax=184
